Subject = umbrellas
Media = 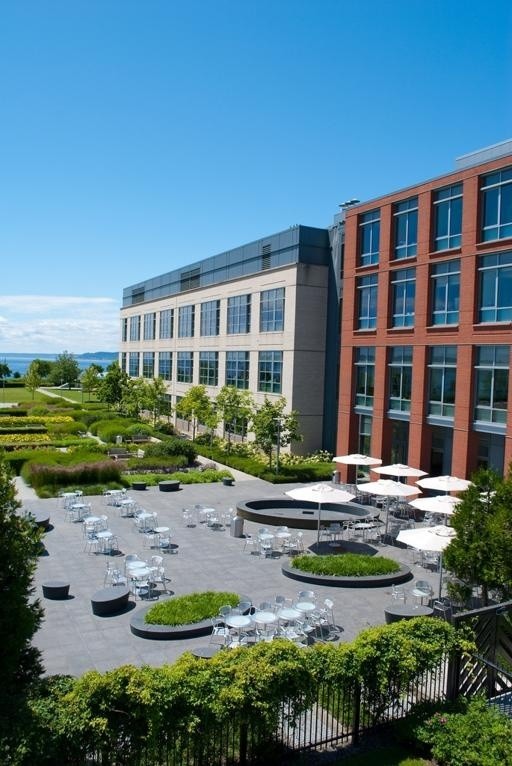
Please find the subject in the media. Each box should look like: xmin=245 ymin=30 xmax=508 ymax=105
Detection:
xmin=414 ymin=475 xmax=477 ymax=496
xmin=370 ymin=463 xmax=429 ymax=509
xmin=284 ymin=484 xmax=356 ymax=549
xmin=332 ymin=454 xmax=382 ymax=485
xmin=356 ymin=479 xmax=423 ymax=543
xmin=395 ymin=524 xmax=458 ymax=602
xmin=408 ymin=495 xmax=465 ymax=527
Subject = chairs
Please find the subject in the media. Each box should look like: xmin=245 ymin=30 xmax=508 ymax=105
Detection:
xmin=321 ymin=485 xmax=450 ymax=573
xmin=209 ymin=591 xmax=339 ymax=653
xmin=58 ymin=488 xmax=171 ymax=600
xmin=243 ymin=526 xmax=304 ymax=559
xmin=392 ymin=581 xmax=434 ymax=606
xmin=182 ymin=504 xmax=235 ymax=531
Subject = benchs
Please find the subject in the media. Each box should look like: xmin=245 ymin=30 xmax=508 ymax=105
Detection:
xmin=107 ymin=448 xmax=128 ymax=458
